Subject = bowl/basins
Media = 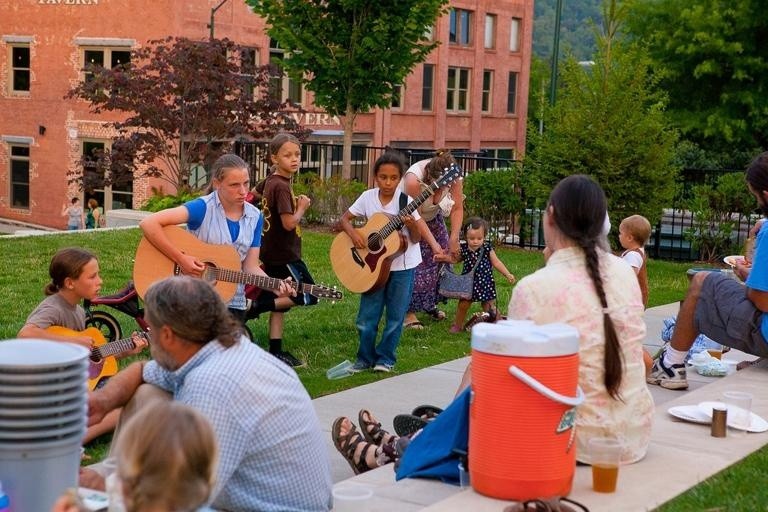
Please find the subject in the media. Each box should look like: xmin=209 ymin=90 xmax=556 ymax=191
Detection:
xmin=0 ymin=338 xmax=90 ymax=512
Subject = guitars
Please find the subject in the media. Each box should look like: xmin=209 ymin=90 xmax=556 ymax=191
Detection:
xmin=330 ymin=163 xmax=462 ymax=293
xmin=132 ymin=226 xmax=343 ymax=305
xmin=43 ymin=326 xmax=150 ymax=392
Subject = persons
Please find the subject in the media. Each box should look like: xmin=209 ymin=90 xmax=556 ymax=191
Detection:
xmin=329 ymin=175 xmax=658 ymax=478
xmin=16 ymin=247 xmax=149 ymax=447
xmin=390 ymin=357 xmax=474 ymax=437
xmin=51 ymin=400 xmax=217 ymax=511
xmin=430 ymin=218 xmax=516 ymax=336
xmin=615 ymin=212 xmax=653 ymax=311
xmin=337 ymin=153 xmax=425 ymax=374
xmin=392 ymin=147 xmax=465 ymax=332
xmin=641 ymin=150 xmax=767 ymax=390
xmin=138 ymin=153 xmax=297 ymax=337
xmin=246 ymin=133 xmax=313 ymax=370
xmin=78 ymin=275 xmax=334 ymax=511
xmin=84 ymin=198 xmax=99 ymax=229
xmin=61 ymin=196 xmax=82 ymax=230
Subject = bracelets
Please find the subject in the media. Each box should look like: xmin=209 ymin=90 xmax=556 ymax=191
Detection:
xmin=505 ymin=273 xmax=511 ymax=279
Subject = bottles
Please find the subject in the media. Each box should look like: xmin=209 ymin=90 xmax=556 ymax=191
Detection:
xmin=711 ymin=406 xmax=727 ymax=438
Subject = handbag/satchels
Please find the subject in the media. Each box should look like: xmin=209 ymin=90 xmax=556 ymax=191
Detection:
xmin=464 ymin=311 xmax=496 ymax=332
xmin=438 ymin=266 xmax=475 ymax=300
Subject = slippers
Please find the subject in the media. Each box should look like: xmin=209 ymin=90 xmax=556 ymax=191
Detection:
xmin=503 ymin=496 xmax=590 ymax=512
xmin=406 ymin=321 xmax=424 ymax=330
xmin=425 ymin=307 xmax=446 ymax=321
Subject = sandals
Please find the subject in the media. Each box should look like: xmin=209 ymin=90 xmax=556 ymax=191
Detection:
xmin=359 ymin=409 xmax=397 ymax=450
xmin=412 ymin=405 xmax=444 ymax=420
xmin=332 ymin=416 xmax=379 ymax=475
xmin=393 ymin=415 xmax=431 ymax=438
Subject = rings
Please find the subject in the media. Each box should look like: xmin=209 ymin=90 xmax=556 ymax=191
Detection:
xmin=455 ymin=247 xmax=459 ymax=251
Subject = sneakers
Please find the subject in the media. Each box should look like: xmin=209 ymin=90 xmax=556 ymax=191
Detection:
xmin=275 ymin=351 xmax=305 ymax=367
xmin=374 ymin=361 xmax=394 ymax=372
xmin=347 ymin=360 xmax=369 ymax=373
xmin=647 ymin=351 xmax=689 ymax=390
xmin=450 ymin=325 xmax=461 ymax=334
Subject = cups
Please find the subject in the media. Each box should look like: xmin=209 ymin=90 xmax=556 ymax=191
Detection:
xmin=326 ymin=360 xmax=355 ymax=381
xmin=706 ymin=338 xmax=723 ymax=362
xmin=458 ymin=464 xmax=468 ymax=490
xmin=588 ymin=436 xmax=623 ymax=494
xmin=723 ymin=390 xmax=752 ymax=437
xmin=332 ymin=486 xmax=373 ymax=512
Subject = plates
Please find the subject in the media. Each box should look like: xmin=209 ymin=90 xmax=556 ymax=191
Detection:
xmin=724 ymin=254 xmax=751 ymax=270
xmin=668 ymin=401 xmax=767 ymax=432
